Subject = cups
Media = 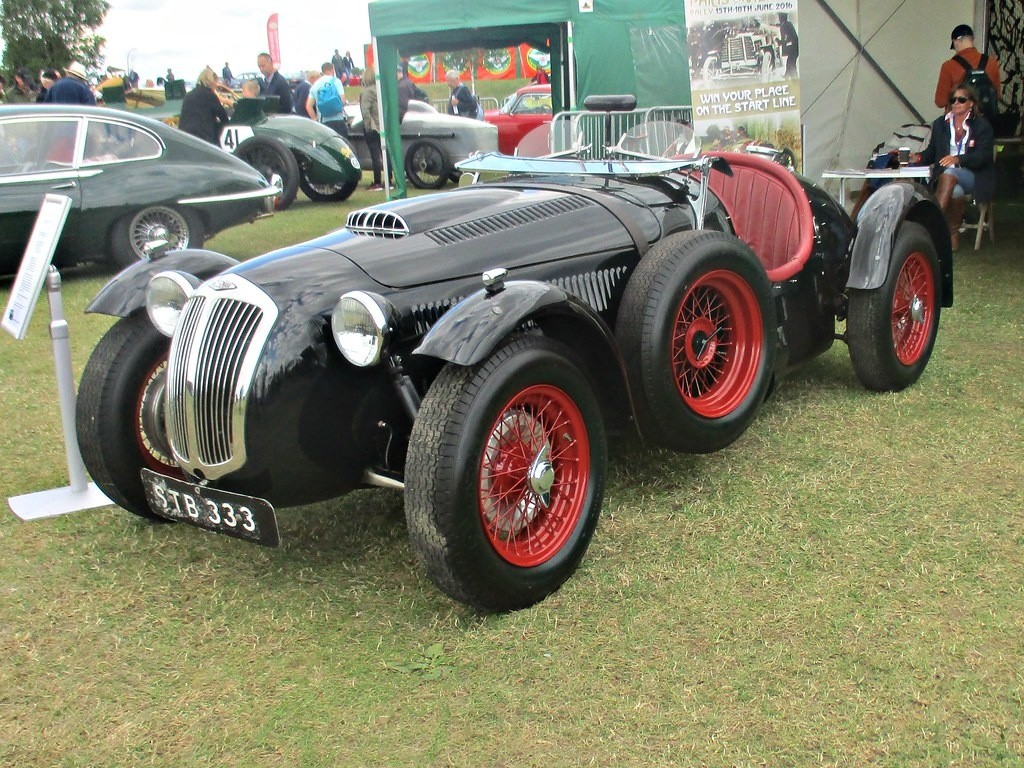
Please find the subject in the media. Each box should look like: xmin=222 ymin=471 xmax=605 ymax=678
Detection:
xmin=899 ymin=147 xmax=910 ymax=165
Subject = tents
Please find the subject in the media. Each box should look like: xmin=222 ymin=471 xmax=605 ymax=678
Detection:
xmin=369 ymin=0 xmax=694 ymax=201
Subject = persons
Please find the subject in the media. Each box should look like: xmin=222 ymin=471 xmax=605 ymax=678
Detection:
xmin=688 ymin=12 xmax=799 ymax=79
xmin=258 ymin=53 xmax=293 ymax=116
xmin=230 ymin=81 xmax=261 ymax=123
xmin=343 ymin=51 xmax=354 ymax=87
xmin=166 ymin=69 xmax=175 ymax=82
xmin=306 ymin=62 xmax=349 ymax=144
xmin=531 ymin=67 xmax=550 ymax=86
xmin=0 ymin=62 xmax=95 ymax=105
xmin=896 ymin=87 xmax=995 ymax=253
xmin=395 ymin=64 xmax=429 ymax=124
xmin=661 ymin=118 xmax=693 ymax=158
xmin=96 ymin=66 xmax=140 ymax=148
xmin=332 ymin=49 xmax=343 ymax=79
xmin=446 ymin=70 xmax=477 ymax=119
xmin=360 ymin=67 xmax=394 ymax=190
xmin=178 ymin=66 xmax=230 ymax=146
xmin=710 ymin=126 xmax=751 ymax=151
xmin=223 ymin=62 xmax=235 ymax=87
xmin=293 ymin=69 xmax=320 ymax=117
xmin=935 ymin=24 xmax=1001 ymax=120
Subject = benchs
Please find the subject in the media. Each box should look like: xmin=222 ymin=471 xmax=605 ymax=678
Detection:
xmin=669 ymin=151 xmax=815 ymax=282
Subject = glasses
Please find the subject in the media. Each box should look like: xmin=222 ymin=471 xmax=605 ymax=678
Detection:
xmin=951 ymin=97 xmax=971 ymax=104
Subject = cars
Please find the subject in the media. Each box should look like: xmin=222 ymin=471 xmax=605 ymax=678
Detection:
xmin=73 ymin=95 xmax=954 ymax=614
xmin=483 ymin=83 xmax=552 ymax=156
xmin=97 ymin=79 xmax=362 ymax=211
xmin=0 ymin=103 xmax=280 ymax=276
xmin=340 ymin=100 xmax=498 ymax=189
xmin=230 ymin=73 xmax=258 ymax=91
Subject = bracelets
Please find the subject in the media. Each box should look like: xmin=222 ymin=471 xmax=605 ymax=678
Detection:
xmin=917 ymin=152 xmax=921 ymax=163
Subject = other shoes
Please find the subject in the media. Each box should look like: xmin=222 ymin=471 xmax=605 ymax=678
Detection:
xmin=366 ymin=182 xmax=383 ymax=191
xmin=381 ymin=180 xmax=394 ymax=190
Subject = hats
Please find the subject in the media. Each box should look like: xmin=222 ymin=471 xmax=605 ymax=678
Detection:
xmin=778 ymin=11 xmax=789 ymax=17
xmin=63 ymin=61 xmax=89 ymax=81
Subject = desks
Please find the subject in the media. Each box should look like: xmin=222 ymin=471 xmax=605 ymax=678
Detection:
xmin=821 ymin=165 xmax=932 ymax=186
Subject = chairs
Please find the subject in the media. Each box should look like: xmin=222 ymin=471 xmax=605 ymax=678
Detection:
xmin=263 ymin=96 xmax=280 ymax=114
xmin=230 ymin=97 xmax=266 ymax=124
xmin=40 ymin=134 xmax=76 ymax=171
xmin=948 ymin=144 xmax=999 ymax=251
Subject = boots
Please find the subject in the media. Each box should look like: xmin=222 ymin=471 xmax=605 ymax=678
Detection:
xmin=936 ymin=173 xmax=959 ymax=214
xmin=950 ymin=198 xmax=965 ymax=251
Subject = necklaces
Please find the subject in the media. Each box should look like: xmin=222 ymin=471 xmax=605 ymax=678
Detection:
xmin=955 ymin=115 xmax=964 ymax=133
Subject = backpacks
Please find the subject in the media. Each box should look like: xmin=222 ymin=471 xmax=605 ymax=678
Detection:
xmin=952 ymin=53 xmax=999 ymax=127
xmin=316 ymin=77 xmax=344 ymax=117
xmin=455 ymin=84 xmax=484 ymax=122
xmin=403 ymin=78 xmax=429 ymax=104
